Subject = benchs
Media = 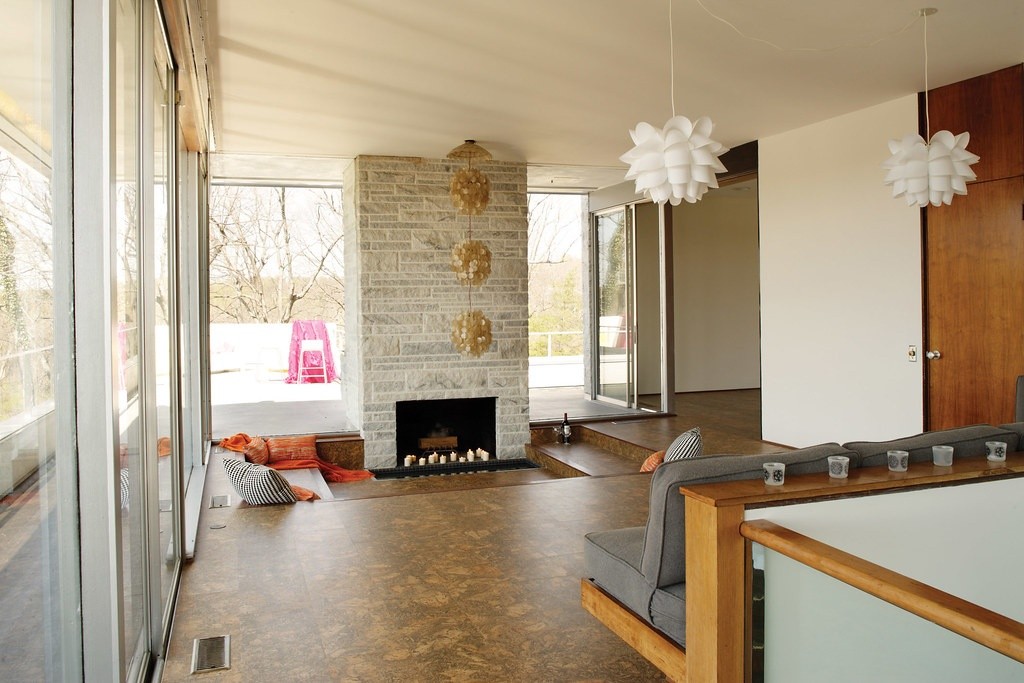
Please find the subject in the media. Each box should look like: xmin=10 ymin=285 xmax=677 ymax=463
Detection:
xmin=580 ymin=375 xmax=1024 ymax=683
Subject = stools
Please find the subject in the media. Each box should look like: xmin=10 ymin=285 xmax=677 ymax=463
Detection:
xmin=296 ymin=340 xmax=328 ymax=383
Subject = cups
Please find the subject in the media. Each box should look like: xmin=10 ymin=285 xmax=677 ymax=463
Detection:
xmin=932 ymin=446 xmax=953 ymax=467
xmin=887 ymin=451 xmax=908 ymax=471
xmin=763 ymin=463 xmax=786 ymax=485
xmin=828 ymin=456 xmax=850 ymax=478
xmin=985 ymin=441 xmax=1007 ymax=462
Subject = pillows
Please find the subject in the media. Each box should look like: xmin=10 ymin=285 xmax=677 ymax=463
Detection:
xmin=265 ymin=436 xmax=320 ymax=463
xmin=641 ymin=448 xmax=665 ymax=473
xmin=223 ymin=458 xmax=298 ymax=505
xmin=242 ymin=435 xmax=269 ymax=466
xmin=291 ymin=486 xmax=321 ymax=501
xmin=664 ymin=426 xmax=702 ymax=463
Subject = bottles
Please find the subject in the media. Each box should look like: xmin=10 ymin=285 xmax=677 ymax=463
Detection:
xmin=561 ymin=413 xmax=570 ymax=443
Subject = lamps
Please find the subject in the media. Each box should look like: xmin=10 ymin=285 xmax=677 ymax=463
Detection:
xmin=882 ymin=10 xmax=980 ymax=207
xmin=619 ymin=0 xmax=731 ymax=205
xmin=446 ymin=139 xmax=493 ymax=355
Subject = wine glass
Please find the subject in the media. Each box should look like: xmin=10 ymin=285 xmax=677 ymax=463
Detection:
xmin=553 ymin=427 xmax=560 ymax=443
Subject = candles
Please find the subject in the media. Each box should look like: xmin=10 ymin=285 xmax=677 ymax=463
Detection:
xmin=404 ymin=454 xmax=417 ymax=466
xmin=459 ymin=457 xmax=466 ymax=462
xmin=449 ymin=452 xmax=456 ymax=461
xmin=476 ymin=448 xmax=490 ymax=461
xmin=440 ymin=455 xmax=446 ymax=463
xmin=428 ymin=452 xmax=438 ymax=464
xmin=419 ymin=458 xmax=425 ymax=465
xmin=467 ymin=449 xmax=474 ymax=461
